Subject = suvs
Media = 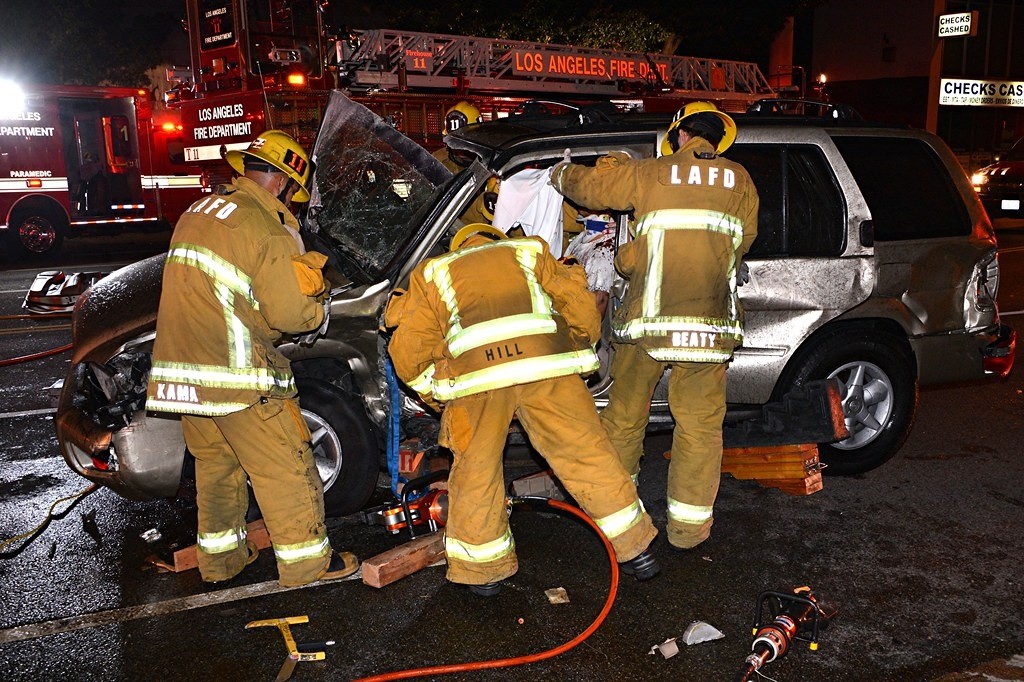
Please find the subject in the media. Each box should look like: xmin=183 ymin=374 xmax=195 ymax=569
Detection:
xmin=52 ymin=94 xmax=1003 ymax=517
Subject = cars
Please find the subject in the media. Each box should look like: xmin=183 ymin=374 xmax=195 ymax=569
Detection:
xmin=971 ymin=137 xmax=1023 ymax=224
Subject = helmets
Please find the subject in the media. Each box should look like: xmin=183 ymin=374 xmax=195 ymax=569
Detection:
xmin=225 ymin=130 xmax=310 ymax=202
xmin=449 ymin=222 xmax=508 ymax=250
xmin=442 ymin=101 xmax=481 ymax=135
xmin=661 ymin=102 xmax=736 ymax=155
xmin=483 ymin=177 xmax=500 ymax=222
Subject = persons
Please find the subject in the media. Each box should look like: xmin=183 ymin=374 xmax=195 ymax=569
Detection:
xmin=140 ymin=130 xmax=358 ymax=586
xmin=451 ymin=176 xmax=503 ymax=238
xmin=423 ymin=100 xmax=487 ymax=177
xmin=382 ymin=221 xmax=663 ymax=595
xmin=548 ymin=101 xmax=765 ymax=553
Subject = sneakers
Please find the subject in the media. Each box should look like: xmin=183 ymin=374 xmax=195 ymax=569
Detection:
xmin=618 ymin=548 xmax=660 ymax=579
xmin=469 ymin=584 xmax=501 ymax=594
xmin=321 ymin=551 xmax=358 ymax=580
xmin=245 ymin=540 xmax=258 ymax=564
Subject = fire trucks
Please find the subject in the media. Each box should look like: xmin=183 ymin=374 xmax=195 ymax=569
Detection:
xmin=2 ymin=0 xmax=787 ymax=264
